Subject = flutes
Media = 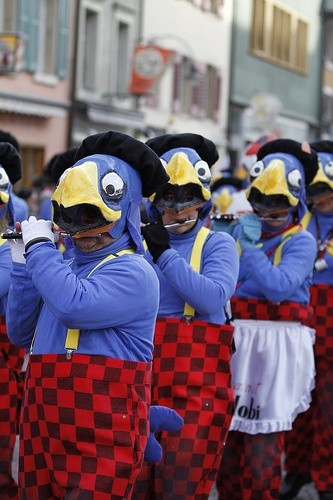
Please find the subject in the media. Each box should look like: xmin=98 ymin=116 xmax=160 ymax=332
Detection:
xmin=210 ymin=215 xmax=288 ymax=220
xmin=163 ymin=218 xmax=197 ymax=229
xmin=0 ymin=228 xmax=102 ymax=239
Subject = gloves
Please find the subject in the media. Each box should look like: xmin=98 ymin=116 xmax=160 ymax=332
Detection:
xmin=237 ymin=212 xmax=262 ymax=248
xmin=213 ymin=216 xmax=239 ymax=236
xmin=140 ymin=213 xmax=170 ymax=263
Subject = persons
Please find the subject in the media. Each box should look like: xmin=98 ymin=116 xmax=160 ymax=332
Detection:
xmin=212 ymin=139 xmax=317 ymax=500
xmin=6 ymin=131 xmax=183 ymax=500
xmin=0 ymin=129 xmax=333 ymax=500
xmin=140 ymin=133 xmax=240 ymax=500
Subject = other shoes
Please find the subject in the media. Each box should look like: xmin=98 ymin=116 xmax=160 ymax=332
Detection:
xmin=280 ymin=472 xmax=309 ymax=499
